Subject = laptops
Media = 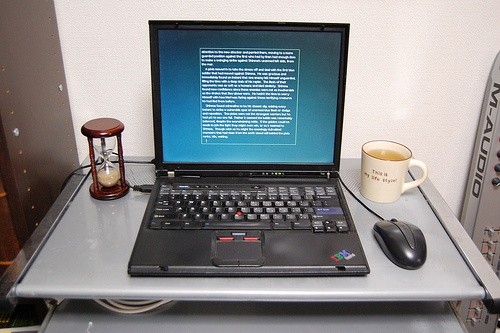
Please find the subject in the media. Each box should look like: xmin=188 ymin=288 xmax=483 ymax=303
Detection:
xmin=129 ymin=19 xmax=370 ymax=276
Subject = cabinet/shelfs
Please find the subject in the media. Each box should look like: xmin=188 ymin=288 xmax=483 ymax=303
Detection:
xmin=1 ymin=0 xmax=81 ymax=333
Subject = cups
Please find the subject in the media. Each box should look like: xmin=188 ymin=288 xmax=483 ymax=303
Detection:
xmin=359 ymin=139 xmax=428 ymax=204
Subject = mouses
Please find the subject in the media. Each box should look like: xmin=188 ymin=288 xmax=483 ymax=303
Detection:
xmin=372 ymin=218 xmax=427 ymax=270
